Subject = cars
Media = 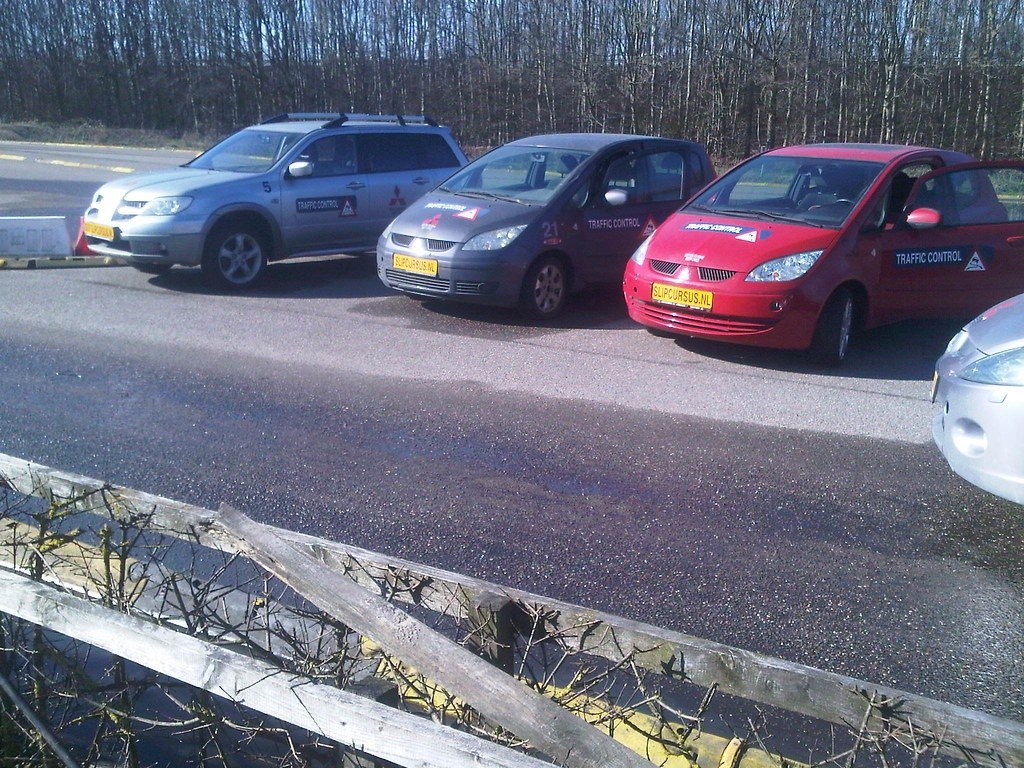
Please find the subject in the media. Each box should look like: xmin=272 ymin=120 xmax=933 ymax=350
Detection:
xmin=930 ymin=294 xmax=1024 ymax=506
xmin=376 ymin=133 xmax=717 ymax=322
xmin=620 ymin=143 xmax=1024 ymax=371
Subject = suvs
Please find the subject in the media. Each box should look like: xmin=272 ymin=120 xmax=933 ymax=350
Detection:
xmin=83 ymin=112 xmax=482 ymax=293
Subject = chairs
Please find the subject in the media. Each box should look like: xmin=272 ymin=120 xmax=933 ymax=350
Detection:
xmin=547 ymin=154 xmax=586 ymax=204
xmin=796 ymin=175 xmax=840 ymax=211
